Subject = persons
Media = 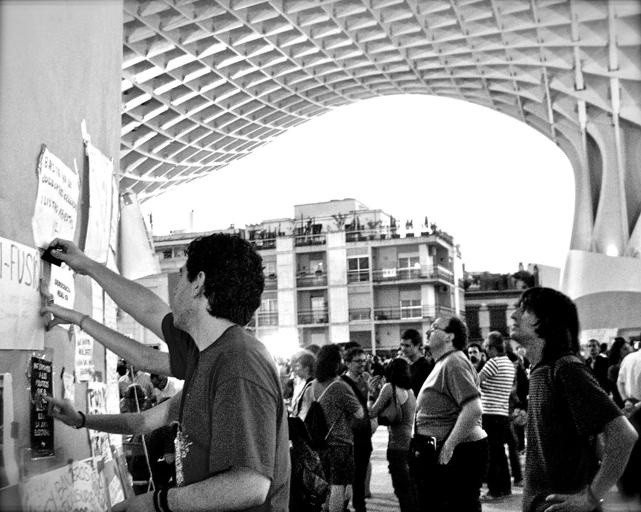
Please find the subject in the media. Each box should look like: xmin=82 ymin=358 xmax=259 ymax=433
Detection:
xmin=274 ymin=287 xmax=640 ymax=511
xmin=40 ymin=238 xmax=201 ymax=382
xmin=115 ymin=357 xmax=179 ymax=497
xmin=41 ymin=233 xmax=295 ymax=510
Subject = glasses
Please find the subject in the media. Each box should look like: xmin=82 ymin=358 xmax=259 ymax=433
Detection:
xmin=352 ymin=358 xmax=367 ymax=363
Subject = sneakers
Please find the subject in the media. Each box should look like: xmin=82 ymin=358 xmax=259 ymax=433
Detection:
xmin=479 ymin=496 xmax=505 ymax=503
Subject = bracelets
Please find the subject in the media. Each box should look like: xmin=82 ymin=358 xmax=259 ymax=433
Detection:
xmin=79 ymin=316 xmax=90 ymax=332
xmin=74 ymin=411 xmax=87 ymax=429
xmin=153 ymin=486 xmax=163 ymax=511
xmin=159 ymin=488 xmax=174 ymax=511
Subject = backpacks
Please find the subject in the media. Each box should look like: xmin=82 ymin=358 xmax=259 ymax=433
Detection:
xmin=283 ymin=371 xmax=294 ymax=400
xmin=299 ymin=378 xmax=345 ymax=451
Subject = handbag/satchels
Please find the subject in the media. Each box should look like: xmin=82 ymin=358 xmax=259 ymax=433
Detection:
xmin=408 ymin=432 xmax=438 ymax=470
xmin=378 ymin=400 xmax=404 ymax=427
